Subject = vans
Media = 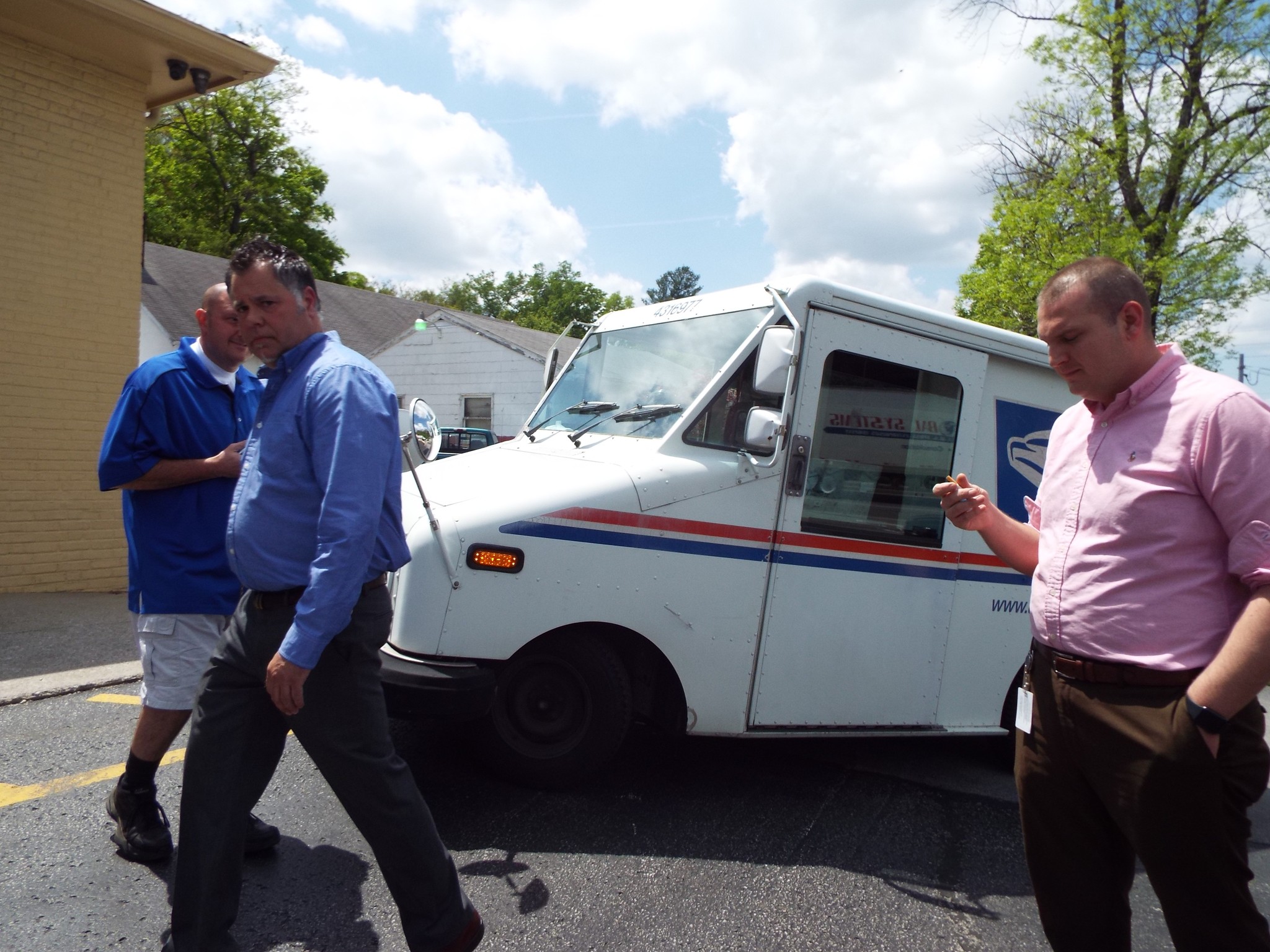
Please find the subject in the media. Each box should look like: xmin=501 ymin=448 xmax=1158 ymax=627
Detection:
xmin=375 ymin=278 xmax=1085 ymax=794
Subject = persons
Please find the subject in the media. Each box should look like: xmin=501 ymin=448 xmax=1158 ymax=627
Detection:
xmin=93 ymin=279 xmax=279 ymax=866
xmin=160 ymin=235 xmax=488 ymax=952
xmin=927 ymin=257 xmax=1270 ymax=952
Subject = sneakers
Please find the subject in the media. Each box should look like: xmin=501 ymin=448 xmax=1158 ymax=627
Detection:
xmin=245 ymin=811 xmax=280 ymax=851
xmin=105 ymin=772 xmax=171 ymax=860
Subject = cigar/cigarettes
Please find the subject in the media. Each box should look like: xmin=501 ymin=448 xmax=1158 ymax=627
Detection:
xmin=945 ymin=475 xmax=963 ymax=489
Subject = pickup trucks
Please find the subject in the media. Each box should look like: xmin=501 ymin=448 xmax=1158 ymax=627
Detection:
xmin=434 ymin=427 xmax=499 ymax=462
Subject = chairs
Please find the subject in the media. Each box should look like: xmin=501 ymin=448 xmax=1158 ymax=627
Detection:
xmin=470 ymin=440 xmax=484 ymax=450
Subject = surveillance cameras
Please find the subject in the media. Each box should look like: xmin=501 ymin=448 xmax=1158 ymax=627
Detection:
xmin=167 ymin=59 xmax=188 ymax=81
xmin=190 ymin=68 xmax=211 ymax=95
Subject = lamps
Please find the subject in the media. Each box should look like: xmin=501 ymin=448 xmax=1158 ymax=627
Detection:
xmin=414 ymin=311 xmax=442 ymax=338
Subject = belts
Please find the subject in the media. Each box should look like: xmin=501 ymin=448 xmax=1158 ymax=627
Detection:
xmin=1032 ymin=641 xmax=1201 ymax=687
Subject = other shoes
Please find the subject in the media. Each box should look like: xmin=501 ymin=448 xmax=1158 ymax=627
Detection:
xmin=447 ymin=911 xmax=485 ymax=952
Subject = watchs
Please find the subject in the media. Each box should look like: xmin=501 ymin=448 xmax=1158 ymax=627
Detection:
xmin=1180 ymin=691 xmax=1236 ymax=737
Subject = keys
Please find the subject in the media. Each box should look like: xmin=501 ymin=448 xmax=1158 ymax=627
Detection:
xmin=1015 ymin=648 xmax=1034 ymax=735
